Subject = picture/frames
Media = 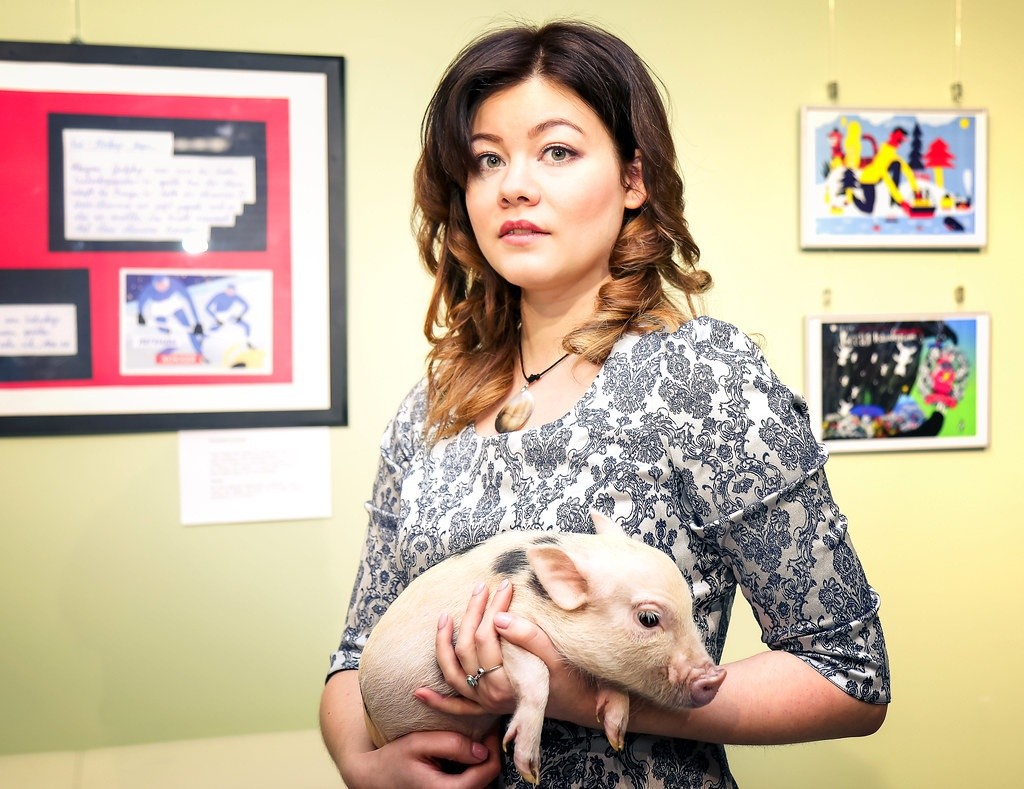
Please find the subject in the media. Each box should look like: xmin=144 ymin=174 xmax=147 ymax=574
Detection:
xmin=799 ymin=103 xmax=986 ymax=250
xmin=801 ymin=312 xmax=990 ymax=453
xmin=0 ymin=36 xmax=346 ymax=435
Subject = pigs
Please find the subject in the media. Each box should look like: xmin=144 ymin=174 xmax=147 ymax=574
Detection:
xmin=358 ymin=504 xmax=727 ymax=784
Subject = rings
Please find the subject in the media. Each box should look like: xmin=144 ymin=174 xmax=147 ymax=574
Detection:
xmin=466 ymin=674 xmax=482 ymax=687
xmin=477 ymin=663 xmax=503 ymax=677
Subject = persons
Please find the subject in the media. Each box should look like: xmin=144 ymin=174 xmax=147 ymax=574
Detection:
xmin=320 ymin=17 xmax=890 ymax=789
